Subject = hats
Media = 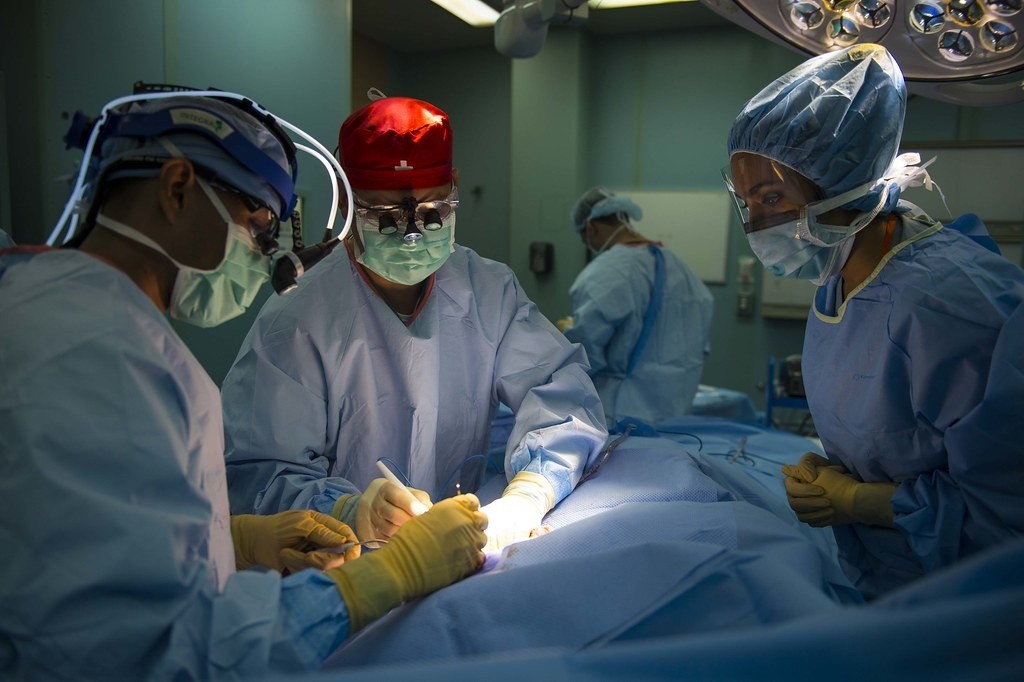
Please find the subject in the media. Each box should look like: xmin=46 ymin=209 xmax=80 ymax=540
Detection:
xmin=337 ymin=97 xmax=454 ymax=192
xmin=98 ymin=95 xmax=290 ymax=226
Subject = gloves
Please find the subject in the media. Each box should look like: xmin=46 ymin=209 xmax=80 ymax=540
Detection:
xmin=324 ymin=494 xmax=487 ymax=633
xmin=780 ymin=451 xmax=898 ymax=528
xmin=227 ymin=510 xmax=360 ymax=575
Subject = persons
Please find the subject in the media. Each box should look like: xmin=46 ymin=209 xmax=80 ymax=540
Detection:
xmin=557 ymin=185 xmax=715 ymax=430
xmin=0 ymin=83 xmax=487 ymax=682
xmin=720 ymin=44 xmax=1024 ymax=604
xmin=220 ymin=97 xmax=609 ymax=556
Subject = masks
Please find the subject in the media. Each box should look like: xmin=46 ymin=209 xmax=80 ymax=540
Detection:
xmin=745 ymin=198 xmax=874 ymax=287
xmin=347 ymin=200 xmax=455 ymax=285
xmin=148 ymin=176 xmax=275 ymax=328
xmin=586 ymin=227 xmax=603 ymax=261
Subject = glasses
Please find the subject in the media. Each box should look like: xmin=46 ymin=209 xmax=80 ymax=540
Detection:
xmin=355 ymin=202 xmax=454 ymax=227
xmin=208 ymin=181 xmax=274 ymax=241
